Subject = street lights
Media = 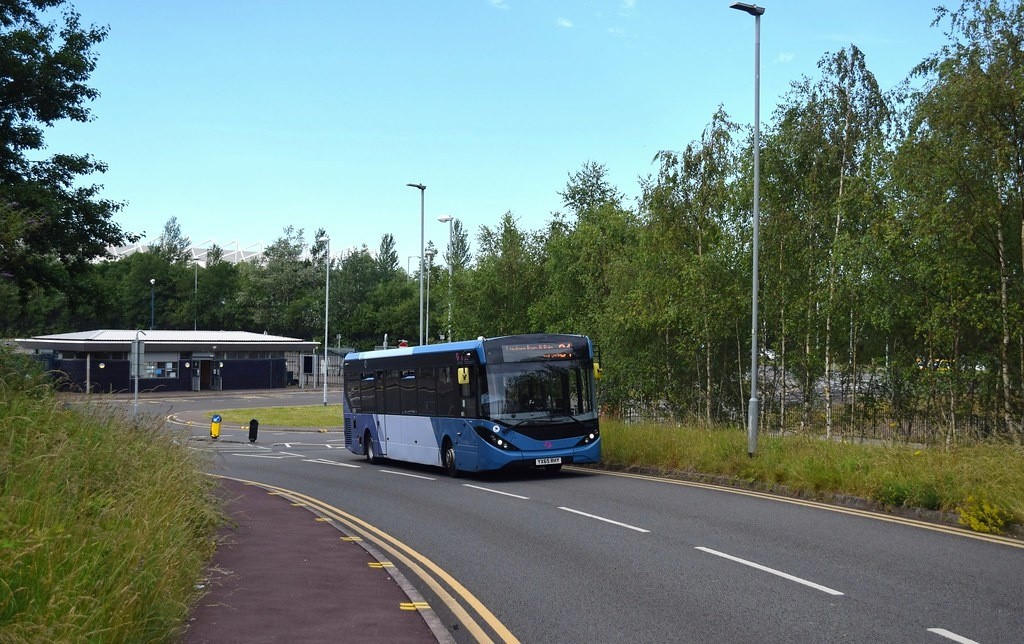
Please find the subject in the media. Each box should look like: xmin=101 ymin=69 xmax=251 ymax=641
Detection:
xmin=437 ymin=213 xmax=455 ymax=343
xmin=318 ymin=237 xmax=329 ymax=406
xmin=407 ymin=255 xmax=415 ymax=280
xmin=424 ymin=251 xmax=435 ymax=344
xmin=405 ymin=181 xmax=424 ymax=346
xmin=729 ymin=0 xmax=760 ymax=457
xmin=193 ymin=256 xmax=200 ymax=329
xmin=149 ymin=278 xmax=157 ymax=330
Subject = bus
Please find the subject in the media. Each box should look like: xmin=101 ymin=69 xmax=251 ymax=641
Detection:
xmin=343 ymin=333 xmax=605 ymax=475
